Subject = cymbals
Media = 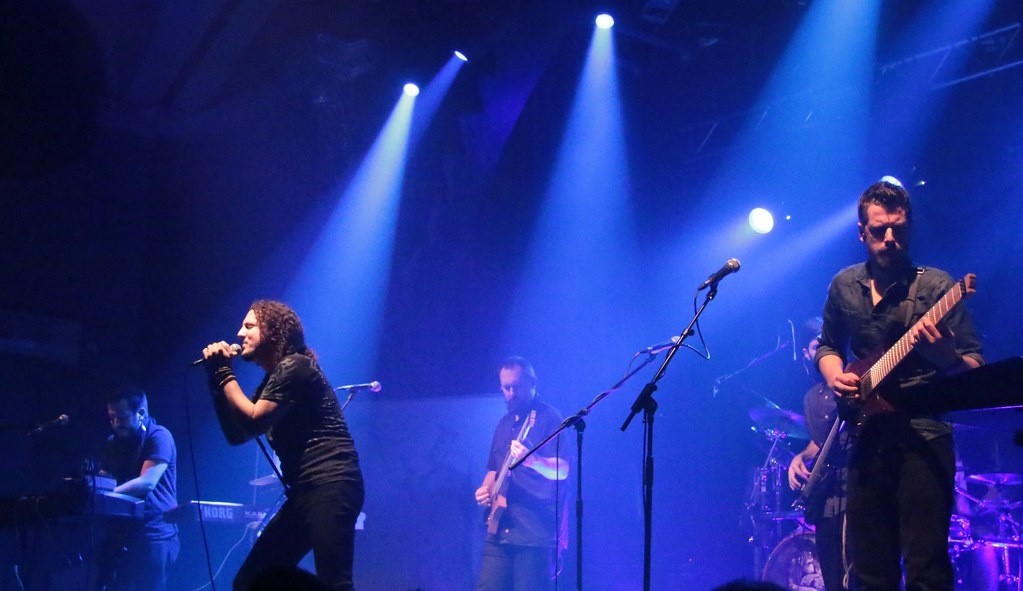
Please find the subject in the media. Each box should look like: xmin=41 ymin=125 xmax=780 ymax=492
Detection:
xmin=748 ymin=407 xmax=810 ymax=440
xmin=965 ymin=473 xmax=1023 ymax=485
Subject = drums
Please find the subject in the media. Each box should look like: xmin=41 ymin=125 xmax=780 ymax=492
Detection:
xmin=760 ymin=532 xmax=825 ymax=591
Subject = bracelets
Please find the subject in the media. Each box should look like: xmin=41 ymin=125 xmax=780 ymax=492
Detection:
xmin=213 ymin=366 xmax=236 ymax=388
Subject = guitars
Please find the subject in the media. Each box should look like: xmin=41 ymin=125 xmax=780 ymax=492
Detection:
xmin=791 ymin=412 xmax=842 ymax=526
xmin=482 ymin=408 xmax=537 ymax=535
xmin=801 ymin=271 xmax=978 ymax=459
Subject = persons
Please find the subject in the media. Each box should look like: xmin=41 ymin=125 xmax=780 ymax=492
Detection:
xmin=96 ymin=385 xmax=181 ymax=591
xmin=202 ymin=300 xmax=366 ymax=591
xmin=788 ymin=316 xmax=854 ymax=591
xmin=474 ymin=356 xmax=570 ymax=591
xmin=813 ymin=182 xmax=985 ymax=591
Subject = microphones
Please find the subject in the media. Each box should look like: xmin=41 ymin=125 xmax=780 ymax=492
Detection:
xmin=337 ymin=380 xmax=382 ymax=392
xmin=27 ymin=414 xmax=69 ymax=437
xmin=192 ymin=343 xmax=242 ymax=367
xmin=787 ymin=320 xmax=797 ymax=361
xmin=638 ymin=336 xmax=681 ymax=354
xmin=698 ymin=257 xmax=742 ymax=292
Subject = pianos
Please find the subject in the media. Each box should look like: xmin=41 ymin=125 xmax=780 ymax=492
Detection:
xmin=162 ymin=499 xmax=282 ymax=525
